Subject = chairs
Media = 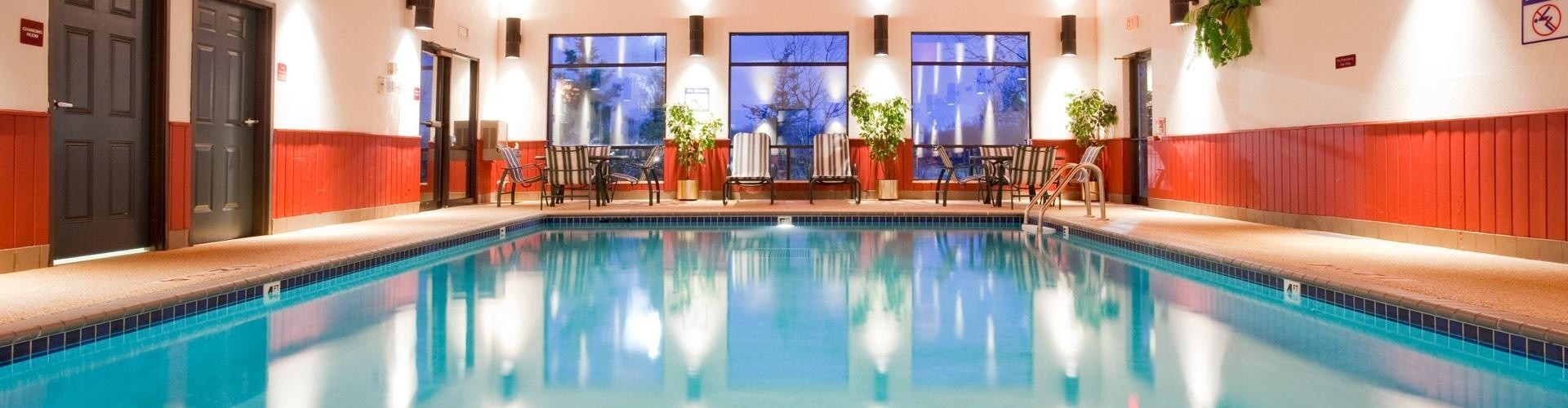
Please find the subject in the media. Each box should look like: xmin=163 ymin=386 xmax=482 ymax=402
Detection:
xmin=931 ymin=143 xmax=1105 ymax=209
xmin=721 ymin=131 xmax=862 ymax=206
xmin=494 ymin=143 xmax=666 ymax=207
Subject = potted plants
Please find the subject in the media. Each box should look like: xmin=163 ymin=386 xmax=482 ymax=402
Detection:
xmin=1064 ymin=86 xmax=1119 ymax=202
xmin=661 ymin=102 xmax=725 ymax=202
xmin=845 ymin=84 xmax=915 ymax=201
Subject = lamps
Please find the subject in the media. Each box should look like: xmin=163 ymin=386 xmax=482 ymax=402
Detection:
xmin=406 ymin=0 xmax=435 ymax=30
xmin=688 ymin=15 xmax=704 ymax=58
xmin=504 ymin=17 xmax=522 ymax=61
xmin=1169 ymin=0 xmax=1200 ymax=26
xmin=874 ymin=13 xmax=889 ymax=56
xmin=1060 ymin=14 xmax=1077 ymax=56
